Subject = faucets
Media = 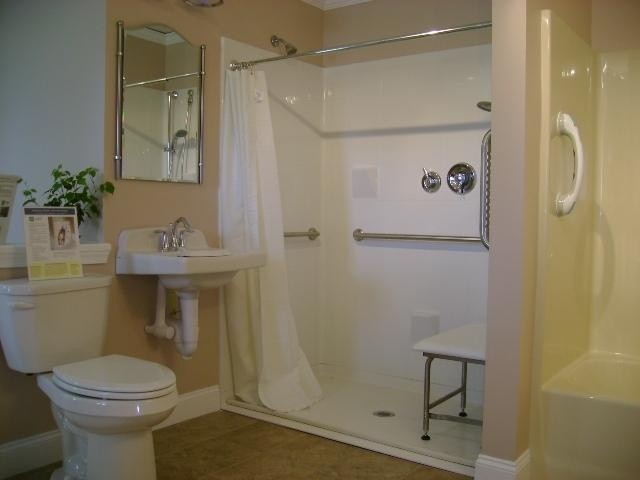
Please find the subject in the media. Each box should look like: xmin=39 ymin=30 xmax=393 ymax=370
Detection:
xmin=168 ymin=216 xmax=192 ymax=249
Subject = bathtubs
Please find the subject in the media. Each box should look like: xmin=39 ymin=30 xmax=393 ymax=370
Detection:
xmin=538 ymin=349 xmax=640 ymax=479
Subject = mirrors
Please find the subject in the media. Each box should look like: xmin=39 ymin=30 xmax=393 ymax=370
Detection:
xmin=113 ymin=19 xmax=206 ymax=184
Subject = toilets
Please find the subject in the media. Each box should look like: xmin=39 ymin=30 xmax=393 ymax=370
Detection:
xmin=0 ymin=272 xmax=179 ymax=480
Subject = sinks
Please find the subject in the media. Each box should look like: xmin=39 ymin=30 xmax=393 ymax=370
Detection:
xmin=116 ymin=225 xmax=266 ymax=289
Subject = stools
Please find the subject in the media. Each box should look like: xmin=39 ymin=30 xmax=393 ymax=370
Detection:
xmin=413 ymin=319 xmax=486 ymax=440
